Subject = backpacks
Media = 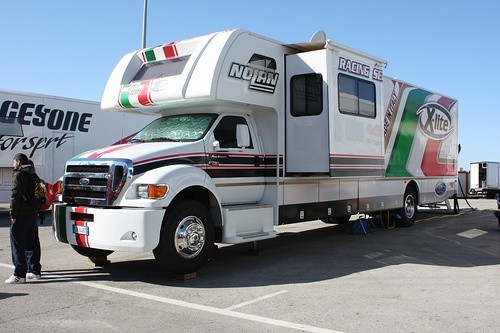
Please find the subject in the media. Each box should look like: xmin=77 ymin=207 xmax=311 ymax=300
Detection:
xmin=18 ymin=171 xmax=45 ymax=209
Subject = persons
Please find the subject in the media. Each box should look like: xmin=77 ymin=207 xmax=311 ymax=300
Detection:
xmin=4 ymin=153 xmax=47 ymax=283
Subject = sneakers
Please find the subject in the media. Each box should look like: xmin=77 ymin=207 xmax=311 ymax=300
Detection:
xmin=26 ymin=272 xmax=41 ymax=280
xmin=5 ymin=274 xmax=26 ymax=284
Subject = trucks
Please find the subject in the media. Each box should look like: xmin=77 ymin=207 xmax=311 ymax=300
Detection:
xmin=50 ymin=27 xmax=458 ymax=273
